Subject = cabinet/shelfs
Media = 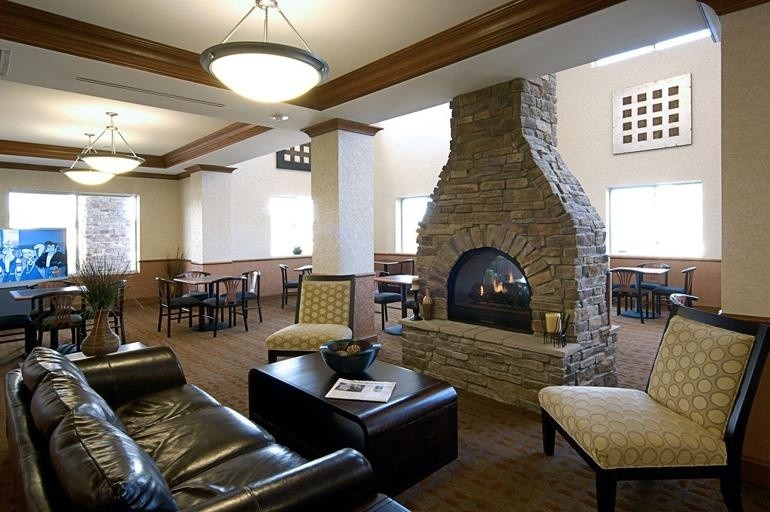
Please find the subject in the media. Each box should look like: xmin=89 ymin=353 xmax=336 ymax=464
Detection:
xmin=422 ymin=287 xmax=434 ymax=320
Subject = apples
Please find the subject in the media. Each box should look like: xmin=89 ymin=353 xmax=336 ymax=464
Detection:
xmin=412 ymin=277 xmax=419 ymax=290
xmin=545 ymin=312 xmax=562 ymax=333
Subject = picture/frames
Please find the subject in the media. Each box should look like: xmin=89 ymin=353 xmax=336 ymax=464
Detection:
xmin=293 ymin=265 xmax=312 ymax=274
xmin=173 ymin=277 xmax=245 ymax=332
xmin=373 ymin=274 xmax=417 ymax=336
xmin=374 ymin=259 xmax=412 ymax=275
xmin=609 ymin=265 xmax=669 ymax=319
xmin=9 ymin=284 xmax=116 ymax=354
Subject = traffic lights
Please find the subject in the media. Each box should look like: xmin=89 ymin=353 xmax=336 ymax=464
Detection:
xmin=21 ymin=346 xmax=181 ymax=512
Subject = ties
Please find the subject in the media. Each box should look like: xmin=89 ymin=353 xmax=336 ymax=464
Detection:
xmin=0 ymin=228 xmax=68 ymax=289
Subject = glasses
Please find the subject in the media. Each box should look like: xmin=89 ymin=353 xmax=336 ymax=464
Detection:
xmin=58 ymin=133 xmax=119 ymax=185
xmin=76 ymin=112 xmax=146 ymax=174
xmin=199 ymin=0 xmax=329 ymax=104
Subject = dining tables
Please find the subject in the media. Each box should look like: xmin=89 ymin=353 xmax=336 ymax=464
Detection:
xmin=158 ymin=247 xmax=185 ymax=296
xmin=69 ymin=252 xmax=143 ymax=358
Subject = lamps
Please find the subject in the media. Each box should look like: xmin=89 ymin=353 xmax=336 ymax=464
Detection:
xmin=264 ymin=273 xmax=357 ymax=362
xmin=220 ymin=269 xmax=263 ymax=324
xmin=372 ymin=285 xmax=403 ymax=331
xmin=636 ymin=261 xmax=670 ymax=318
xmin=651 ymin=267 xmax=696 ymax=319
xmin=610 ymin=267 xmax=649 ymax=324
xmin=25 ymin=279 xmax=79 ymax=348
xmin=536 ymin=301 xmax=769 ymax=510
xmin=278 ymin=263 xmax=300 ymax=309
xmin=79 ymin=279 xmax=128 ymax=351
xmin=399 ymin=261 xmax=414 ymax=275
xmin=176 ymin=271 xmax=212 ymax=327
xmin=23 ymin=291 xmax=87 ymax=356
xmin=610 ymin=284 xmax=632 ymax=311
xmin=202 ymin=276 xmax=248 ymax=338
xmin=155 ymin=277 xmax=203 ymax=338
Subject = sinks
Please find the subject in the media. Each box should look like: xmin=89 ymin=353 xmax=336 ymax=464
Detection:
xmin=6 ymin=344 xmax=376 ymax=512
